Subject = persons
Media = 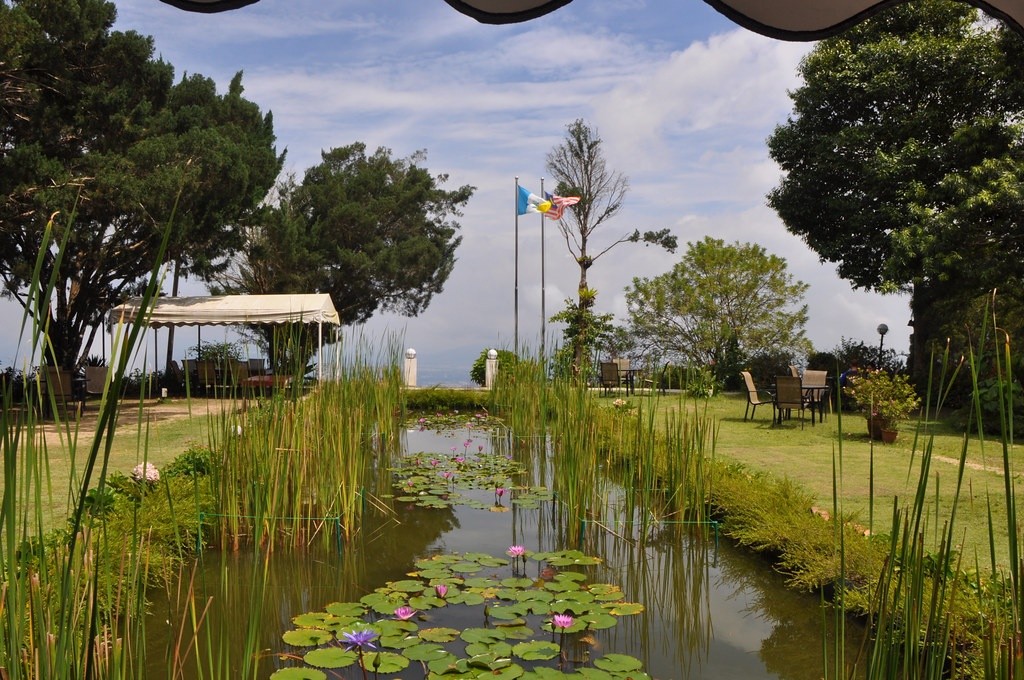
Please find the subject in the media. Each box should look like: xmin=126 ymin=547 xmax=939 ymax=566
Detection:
xmin=865 ymin=362 xmax=876 ymax=380
xmin=840 ymin=359 xmax=863 ymax=413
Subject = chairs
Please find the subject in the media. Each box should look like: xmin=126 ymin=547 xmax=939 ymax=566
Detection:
xmin=601 ymin=358 xmax=630 ymax=396
xmin=170 ymin=358 xmax=265 ymax=398
xmin=32 ymin=366 xmax=109 ymax=421
xmin=740 ymin=365 xmax=828 ymax=430
xmin=645 ymin=361 xmax=669 ymax=397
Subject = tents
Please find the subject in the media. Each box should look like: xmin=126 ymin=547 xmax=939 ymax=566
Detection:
xmin=109 ymin=289 xmax=341 ymax=394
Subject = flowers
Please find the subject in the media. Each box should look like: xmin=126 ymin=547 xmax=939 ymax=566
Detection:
xmin=842 ymin=366 xmax=922 ymax=431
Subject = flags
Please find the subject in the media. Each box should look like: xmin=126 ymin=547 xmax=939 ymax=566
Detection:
xmin=517 ymin=184 xmax=551 ymax=215
xmin=543 ymin=191 xmax=581 ymax=219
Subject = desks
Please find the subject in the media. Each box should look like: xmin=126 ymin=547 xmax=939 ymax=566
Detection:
xmin=37 ymin=379 xmax=91 ymax=418
xmin=195 ymin=369 xmax=264 ymax=398
xmin=617 ymin=370 xmax=644 ymax=397
xmin=772 ymin=384 xmax=829 ymax=427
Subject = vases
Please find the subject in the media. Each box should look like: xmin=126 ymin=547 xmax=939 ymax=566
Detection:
xmin=881 ymin=430 xmax=898 ymax=444
xmin=868 ymin=418 xmax=889 ymax=440
